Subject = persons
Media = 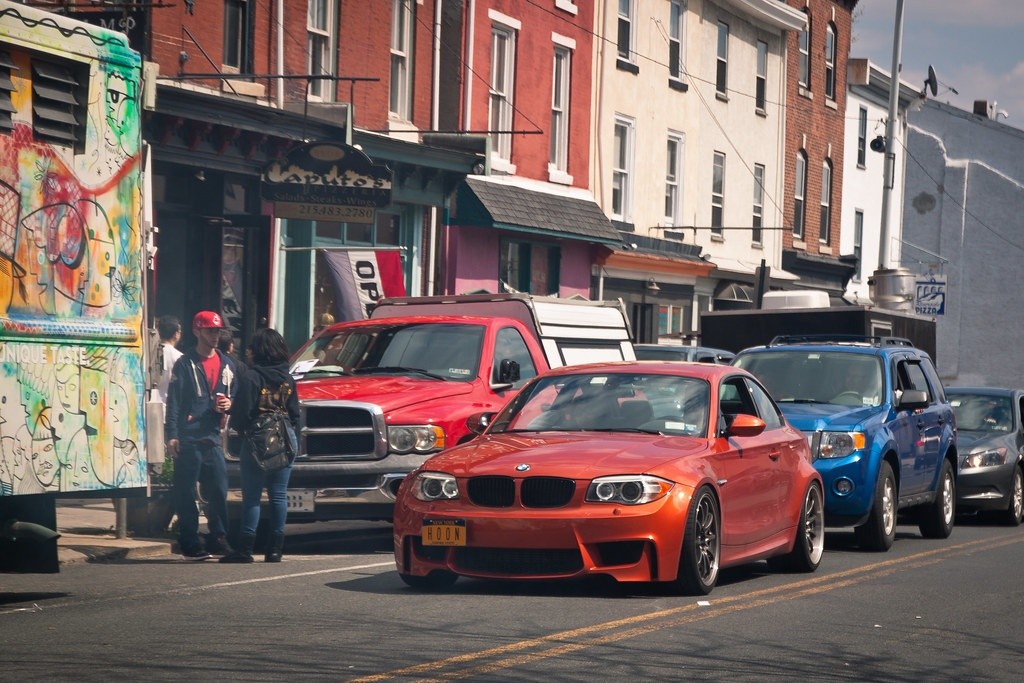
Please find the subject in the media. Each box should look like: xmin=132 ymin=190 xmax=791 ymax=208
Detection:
xmin=681 ymin=384 xmax=706 ymax=432
xmin=154 ymin=311 xmax=345 ymax=565
xmin=570 ymin=394 xmax=606 ymax=429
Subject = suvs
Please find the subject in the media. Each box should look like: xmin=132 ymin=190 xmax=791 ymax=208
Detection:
xmin=711 ymin=331 xmax=958 ymax=552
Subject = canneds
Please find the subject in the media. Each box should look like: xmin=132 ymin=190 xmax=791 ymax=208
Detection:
xmin=216 ymin=393 xmax=225 ymax=413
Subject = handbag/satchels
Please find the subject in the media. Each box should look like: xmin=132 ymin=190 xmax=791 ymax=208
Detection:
xmin=244 ymin=409 xmax=295 ymax=471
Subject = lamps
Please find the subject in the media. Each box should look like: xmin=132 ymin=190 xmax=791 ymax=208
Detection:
xmin=647 ymin=277 xmax=660 ymax=296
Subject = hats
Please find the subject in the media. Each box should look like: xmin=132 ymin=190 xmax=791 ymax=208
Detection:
xmin=193 ymin=311 xmax=222 ymax=330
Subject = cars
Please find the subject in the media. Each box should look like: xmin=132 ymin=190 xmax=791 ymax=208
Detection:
xmin=631 ymin=344 xmax=740 ymax=423
xmin=943 ymin=384 xmax=1024 ymax=526
xmin=382 ymin=355 xmax=828 ymax=599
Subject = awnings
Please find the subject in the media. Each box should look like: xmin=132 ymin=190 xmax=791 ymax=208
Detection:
xmin=465 ymin=177 xmax=624 ymax=246
xmin=712 ymin=279 xmax=780 ymax=303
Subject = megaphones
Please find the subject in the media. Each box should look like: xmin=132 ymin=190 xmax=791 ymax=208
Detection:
xmin=870 ymin=139 xmax=886 ymax=153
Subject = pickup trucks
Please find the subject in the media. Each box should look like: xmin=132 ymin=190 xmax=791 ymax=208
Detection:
xmin=192 ymin=290 xmax=647 ymax=553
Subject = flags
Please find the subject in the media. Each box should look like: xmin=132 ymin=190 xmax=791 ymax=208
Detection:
xmin=325 ymin=244 xmax=404 ymax=324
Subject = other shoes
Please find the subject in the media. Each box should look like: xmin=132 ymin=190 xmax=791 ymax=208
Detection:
xmin=218 ymin=551 xmax=254 ymax=564
xmin=180 ymin=541 xmax=210 ymax=560
xmin=204 ymin=534 xmax=234 ymax=558
xmin=265 ymin=551 xmax=282 ymax=562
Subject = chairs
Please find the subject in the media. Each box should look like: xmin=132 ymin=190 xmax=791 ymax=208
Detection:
xmin=619 ymin=400 xmax=654 ymax=428
